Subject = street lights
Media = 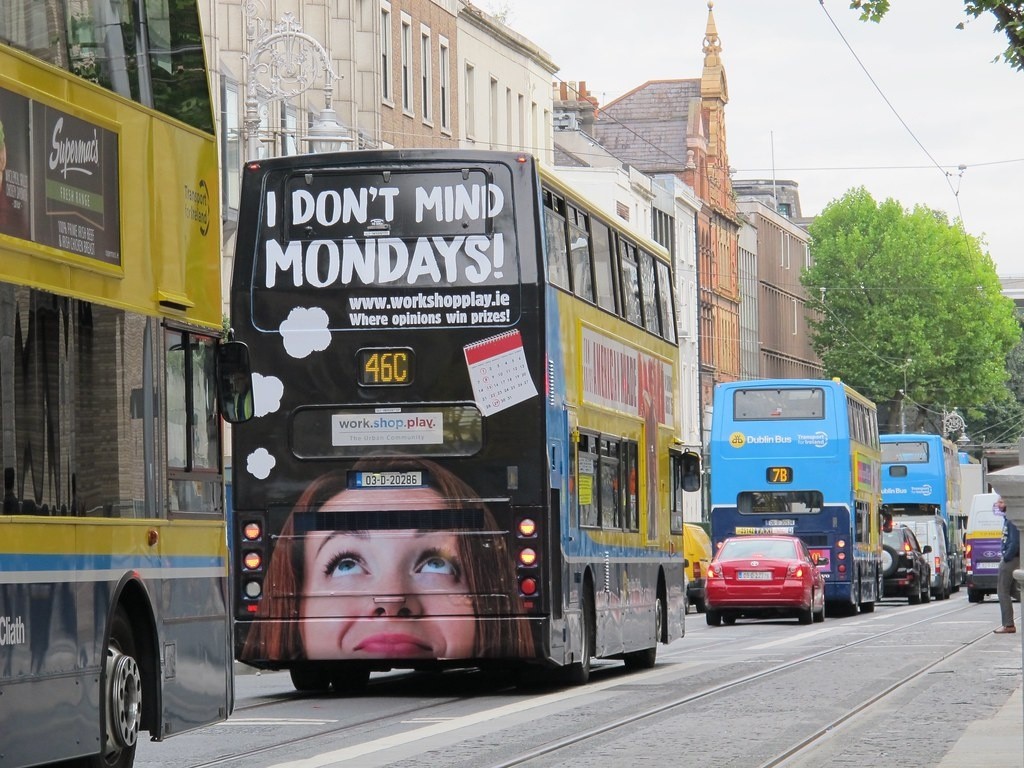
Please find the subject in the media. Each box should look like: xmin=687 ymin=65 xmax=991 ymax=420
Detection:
xmin=238 ymin=30 xmax=356 ymax=161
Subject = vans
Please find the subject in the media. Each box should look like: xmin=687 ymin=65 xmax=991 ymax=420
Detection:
xmin=705 ymin=537 xmax=829 ymax=625
xmin=966 ymin=491 xmax=1023 ymax=601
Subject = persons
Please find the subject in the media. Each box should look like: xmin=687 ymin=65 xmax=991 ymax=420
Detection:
xmin=993 ymin=495 xmax=1020 ymax=633
xmin=243 ymin=448 xmax=536 ymax=659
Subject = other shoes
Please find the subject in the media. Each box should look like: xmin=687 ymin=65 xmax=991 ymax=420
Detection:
xmin=993 ymin=626 xmax=1016 ymax=633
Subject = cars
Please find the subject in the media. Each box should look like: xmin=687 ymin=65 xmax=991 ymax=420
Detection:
xmin=681 ymin=523 xmax=711 ymax=613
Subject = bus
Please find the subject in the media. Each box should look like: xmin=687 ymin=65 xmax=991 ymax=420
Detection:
xmin=221 ymin=149 xmax=701 ymax=696
xmin=876 ymin=433 xmax=964 ymax=590
xmin=704 ymin=376 xmax=895 ymax=612
xmin=1 ymin=43 xmax=234 ymax=762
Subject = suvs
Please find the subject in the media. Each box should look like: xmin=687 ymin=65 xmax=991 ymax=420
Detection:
xmin=881 ymin=525 xmax=941 ymax=601
xmin=890 ymin=513 xmax=955 ymax=600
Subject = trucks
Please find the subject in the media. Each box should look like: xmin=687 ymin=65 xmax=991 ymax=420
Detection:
xmin=955 ymin=452 xmax=991 ymax=542
